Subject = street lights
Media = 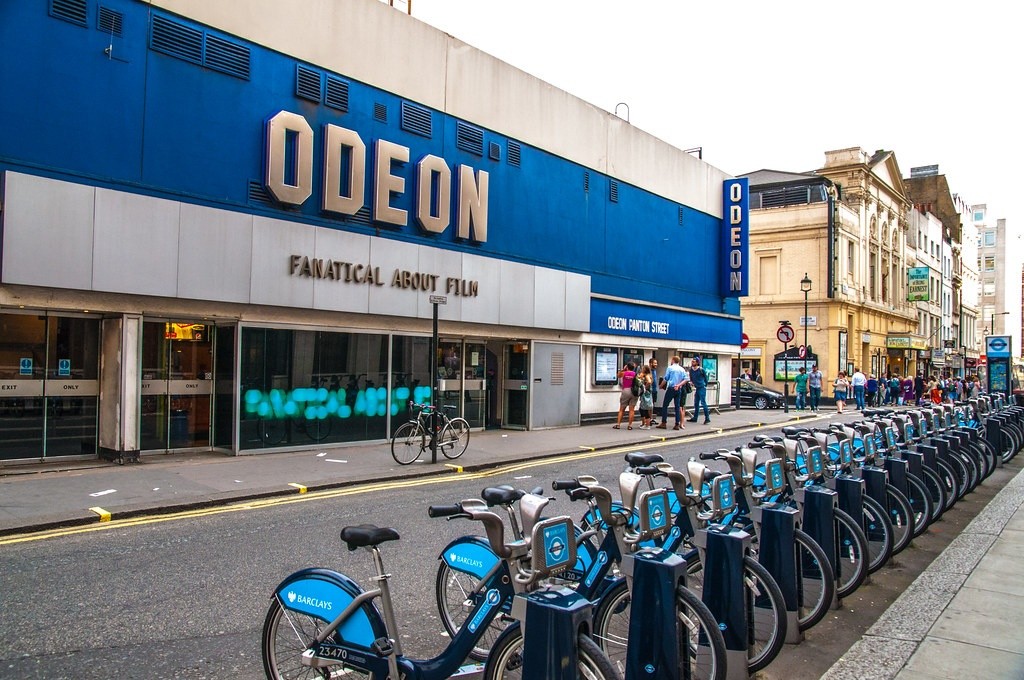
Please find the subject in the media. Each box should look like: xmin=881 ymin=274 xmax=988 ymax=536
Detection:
xmin=799 ymin=272 xmax=812 ymax=374
xmin=990 ymin=311 xmax=1010 ymax=336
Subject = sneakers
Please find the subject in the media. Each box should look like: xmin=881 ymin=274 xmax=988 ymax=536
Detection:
xmin=638 ymin=424 xmax=645 ymax=428
xmin=642 ymin=426 xmax=651 ymax=430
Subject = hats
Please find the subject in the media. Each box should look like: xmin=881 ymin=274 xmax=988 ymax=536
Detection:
xmin=696 ymin=356 xmax=700 ymax=366
xmin=813 ymin=364 xmax=817 ymax=367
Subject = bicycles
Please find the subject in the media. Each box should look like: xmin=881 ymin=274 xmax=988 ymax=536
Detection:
xmin=432 ymin=393 xmax=1024 ymax=680
xmin=390 ymin=400 xmax=471 ymax=466
xmin=259 ymin=486 xmax=625 ymax=680
xmin=256 ymin=387 xmax=332 ymax=447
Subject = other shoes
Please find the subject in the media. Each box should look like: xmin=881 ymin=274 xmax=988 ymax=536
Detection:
xmin=687 ymin=419 xmax=697 ymax=422
xmin=704 ymin=419 xmax=710 ymax=424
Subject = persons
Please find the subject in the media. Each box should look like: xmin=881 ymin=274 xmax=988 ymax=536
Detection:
xmin=687 ymin=357 xmax=710 ymax=424
xmin=792 ymin=367 xmax=809 ymax=412
xmin=756 ymin=371 xmax=762 ymax=384
xmin=638 ymin=365 xmax=653 ymax=430
xmin=741 ymin=370 xmax=753 ymax=380
xmin=613 ymin=363 xmax=638 ymax=430
xmin=656 ymin=355 xmax=690 ymax=430
xmin=649 ymin=358 xmax=659 ymax=426
xmin=809 ymin=364 xmax=823 ymax=412
xmin=832 ymin=367 xmax=980 ymax=413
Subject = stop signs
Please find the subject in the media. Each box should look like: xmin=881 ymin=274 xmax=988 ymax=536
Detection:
xmin=740 ymin=333 xmax=750 ymax=350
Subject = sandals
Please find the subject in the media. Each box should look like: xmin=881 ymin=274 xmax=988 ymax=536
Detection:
xmin=613 ymin=425 xmax=620 ymax=429
xmin=628 ymin=426 xmax=633 ymax=429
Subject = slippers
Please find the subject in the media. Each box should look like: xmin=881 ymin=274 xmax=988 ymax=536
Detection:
xmin=674 ymin=427 xmax=679 ymax=430
xmin=656 ymin=425 xmax=666 ymax=428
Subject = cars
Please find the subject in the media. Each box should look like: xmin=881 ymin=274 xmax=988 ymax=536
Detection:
xmin=731 ymin=377 xmax=786 ymax=411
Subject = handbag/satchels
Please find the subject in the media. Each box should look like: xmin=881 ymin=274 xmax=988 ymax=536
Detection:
xmin=686 ymin=382 xmax=692 ymax=393
xmin=659 ymin=378 xmax=667 ymax=390
xmin=640 ymin=386 xmax=653 ymax=410
xmin=654 ymin=391 xmax=657 ymax=402
xmin=631 ymin=374 xmax=646 ymax=397
